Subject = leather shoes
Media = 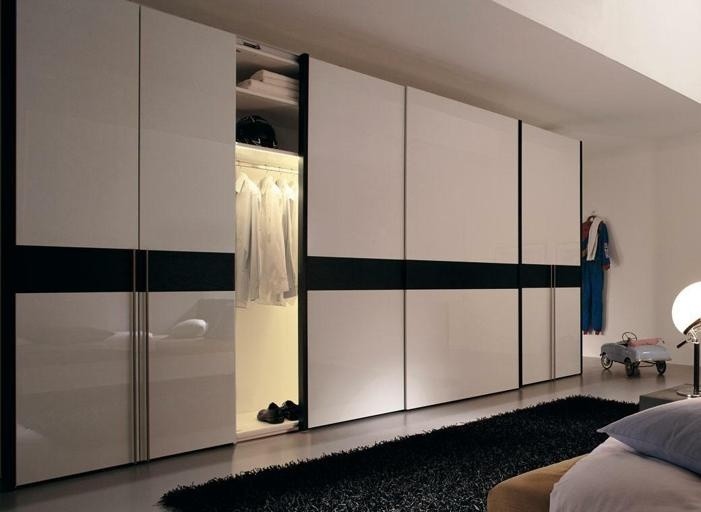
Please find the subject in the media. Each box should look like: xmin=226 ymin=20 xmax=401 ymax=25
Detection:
xmin=257 ymin=400 xmax=298 ymax=424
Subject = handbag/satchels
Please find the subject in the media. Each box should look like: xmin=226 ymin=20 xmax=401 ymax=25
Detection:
xmin=236 ymin=112 xmax=278 ymax=148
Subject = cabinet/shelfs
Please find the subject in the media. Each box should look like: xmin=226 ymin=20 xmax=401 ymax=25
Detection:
xmin=407 ymin=84 xmax=585 ymax=411
xmin=14 ymin=1 xmax=236 ymax=488
xmin=237 ymin=36 xmax=406 ymax=444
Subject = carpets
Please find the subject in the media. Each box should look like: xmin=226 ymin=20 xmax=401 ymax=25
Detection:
xmin=161 ymin=394 xmax=640 ymax=512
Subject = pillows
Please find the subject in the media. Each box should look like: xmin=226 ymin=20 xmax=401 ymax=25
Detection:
xmin=595 ymin=395 xmax=701 ymax=479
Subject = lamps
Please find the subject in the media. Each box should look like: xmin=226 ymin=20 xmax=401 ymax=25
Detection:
xmin=669 ymin=280 xmax=701 ymax=398
